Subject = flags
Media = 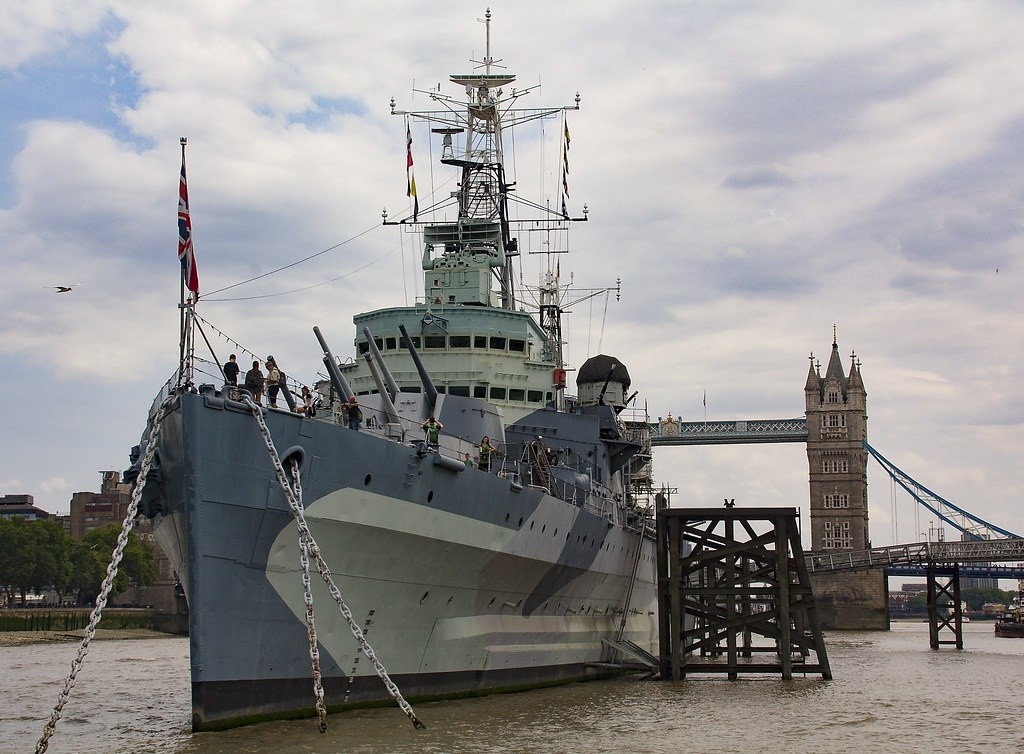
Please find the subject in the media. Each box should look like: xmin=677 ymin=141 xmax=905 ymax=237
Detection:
xmin=405 ymin=122 xmax=420 ymax=225
xmin=561 ymin=119 xmax=571 ymax=219
xmin=177 ymin=159 xmax=199 ymax=304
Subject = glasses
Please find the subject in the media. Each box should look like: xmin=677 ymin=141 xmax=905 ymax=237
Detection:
xmin=265 ymin=365 xmax=272 ymax=369
xmin=302 ymin=391 xmax=306 ymax=394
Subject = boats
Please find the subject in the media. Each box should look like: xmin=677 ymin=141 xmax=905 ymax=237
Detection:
xmin=950 ymin=617 xmax=970 ymax=623
xmin=993 ymin=612 xmax=1024 ymax=639
xmin=141 ymin=0 xmax=673 ymax=732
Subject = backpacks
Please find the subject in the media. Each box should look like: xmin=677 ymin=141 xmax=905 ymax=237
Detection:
xmin=425 ymin=424 xmax=439 ymax=445
xmin=279 ymin=371 xmax=287 ymax=388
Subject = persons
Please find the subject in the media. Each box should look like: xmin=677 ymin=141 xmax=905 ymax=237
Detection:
xmin=341 ymin=395 xmax=361 ymax=432
xmin=462 ymin=435 xmax=495 ymax=472
xmin=222 ymin=353 xmax=280 ymax=409
xmin=420 ymin=417 xmax=443 ymax=452
xmin=298 ymin=386 xmax=313 ymax=419
